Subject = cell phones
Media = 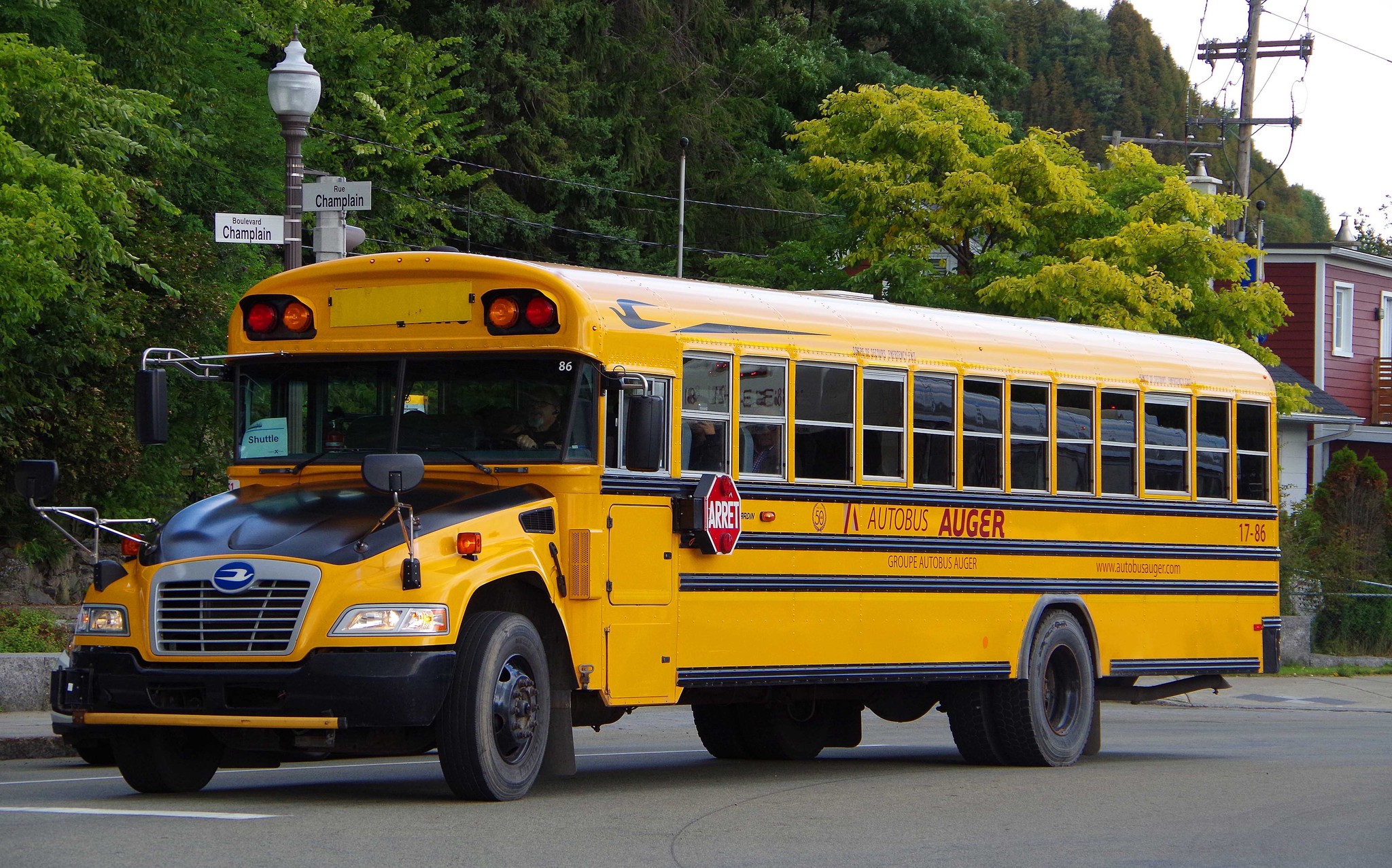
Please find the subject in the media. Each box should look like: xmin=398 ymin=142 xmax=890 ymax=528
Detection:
xmin=698 ymin=403 xmax=708 ymax=422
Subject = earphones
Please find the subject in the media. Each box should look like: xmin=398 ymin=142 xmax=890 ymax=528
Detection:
xmin=552 ymin=410 xmax=559 ymax=415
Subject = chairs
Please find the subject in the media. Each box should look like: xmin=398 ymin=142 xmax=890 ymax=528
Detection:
xmin=739 ymin=428 xmax=755 ymax=473
xmin=680 ymin=420 xmax=692 ymax=470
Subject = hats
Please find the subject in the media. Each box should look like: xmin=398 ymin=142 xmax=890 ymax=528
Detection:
xmin=524 ymin=384 xmax=565 ymax=406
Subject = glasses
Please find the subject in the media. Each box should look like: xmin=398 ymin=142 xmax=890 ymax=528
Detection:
xmin=529 ymin=401 xmax=554 ymax=406
xmin=754 ymin=423 xmax=778 ymax=431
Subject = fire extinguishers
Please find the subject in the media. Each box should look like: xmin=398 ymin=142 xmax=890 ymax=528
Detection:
xmin=327 ymin=419 xmax=345 ymax=451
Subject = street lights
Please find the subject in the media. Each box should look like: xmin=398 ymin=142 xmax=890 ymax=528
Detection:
xmin=265 ymin=36 xmax=321 ymax=455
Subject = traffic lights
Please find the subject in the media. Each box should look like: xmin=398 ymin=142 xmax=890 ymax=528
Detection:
xmin=313 ymin=175 xmax=366 ymax=262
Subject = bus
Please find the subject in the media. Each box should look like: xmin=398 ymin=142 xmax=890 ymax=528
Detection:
xmin=9 ymin=249 xmax=1282 ymax=801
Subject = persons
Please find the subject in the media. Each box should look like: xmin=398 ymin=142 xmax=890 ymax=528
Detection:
xmin=684 ymin=395 xmax=787 ymax=478
xmin=491 ymin=388 xmax=587 ymax=460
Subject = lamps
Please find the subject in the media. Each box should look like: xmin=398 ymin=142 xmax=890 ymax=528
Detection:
xmin=1375 ymin=307 xmax=1384 ymax=321
